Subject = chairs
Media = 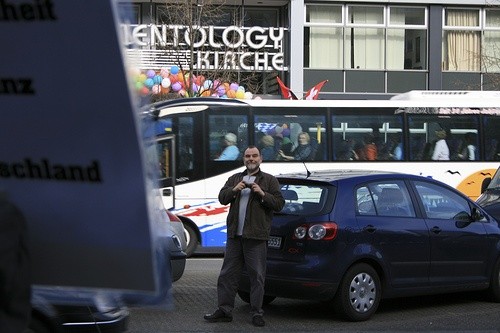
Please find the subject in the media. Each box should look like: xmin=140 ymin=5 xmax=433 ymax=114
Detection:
xmin=378 ymin=188 xmax=410 ymax=214
xmin=280 ymin=189 xmax=302 ymax=211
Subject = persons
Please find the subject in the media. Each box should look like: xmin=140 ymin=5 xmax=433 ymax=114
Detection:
xmin=203 ymin=146 xmax=285 ymax=327
xmin=215 ymin=123 xmax=481 ymax=159
xmin=280 ymin=133 xmax=312 ymax=160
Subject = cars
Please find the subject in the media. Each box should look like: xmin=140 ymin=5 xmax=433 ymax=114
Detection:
xmin=236 ymin=169 xmax=500 ymax=321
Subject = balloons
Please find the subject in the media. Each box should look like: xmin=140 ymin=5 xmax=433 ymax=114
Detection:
xmin=131 ymin=65 xmax=255 ymax=100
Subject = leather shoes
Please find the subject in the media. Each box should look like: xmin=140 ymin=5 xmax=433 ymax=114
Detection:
xmin=252 ymin=315 xmax=265 ymax=327
xmin=204 ymin=308 xmax=233 ymax=322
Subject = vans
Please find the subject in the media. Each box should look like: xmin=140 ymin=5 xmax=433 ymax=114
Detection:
xmin=475 ymin=166 xmax=500 ymax=225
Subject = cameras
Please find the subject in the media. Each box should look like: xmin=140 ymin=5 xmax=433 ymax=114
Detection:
xmin=245 ymin=184 xmax=253 ymax=188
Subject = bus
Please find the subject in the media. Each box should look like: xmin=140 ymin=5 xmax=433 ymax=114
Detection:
xmin=138 ymin=90 xmax=500 ymax=257
